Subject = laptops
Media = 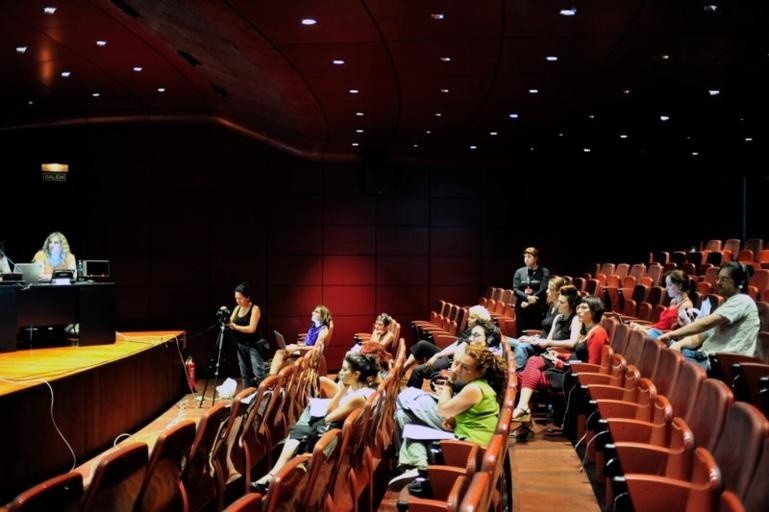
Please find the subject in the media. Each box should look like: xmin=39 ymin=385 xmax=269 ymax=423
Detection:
xmin=13 ymin=263 xmax=41 ymax=281
xmin=39 ymin=268 xmax=75 ymax=283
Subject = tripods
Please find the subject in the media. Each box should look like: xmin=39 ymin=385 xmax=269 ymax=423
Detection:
xmin=199 ymin=330 xmax=224 ymax=407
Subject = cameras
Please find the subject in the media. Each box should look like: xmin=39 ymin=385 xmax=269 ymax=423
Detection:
xmin=216 ymin=306 xmax=231 ymax=324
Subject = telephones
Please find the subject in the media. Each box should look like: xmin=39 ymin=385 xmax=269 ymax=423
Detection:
xmin=51 ymin=269 xmax=75 ymax=282
xmin=1 ymin=273 xmax=27 ymax=281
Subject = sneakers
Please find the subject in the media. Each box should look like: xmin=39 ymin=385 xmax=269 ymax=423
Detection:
xmin=248 ymin=476 xmax=270 ymax=494
xmin=388 ymin=469 xmax=419 ymax=492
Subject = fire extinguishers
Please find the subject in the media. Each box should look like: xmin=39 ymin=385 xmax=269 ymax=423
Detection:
xmin=185 ymin=353 xmax=196 ymax=392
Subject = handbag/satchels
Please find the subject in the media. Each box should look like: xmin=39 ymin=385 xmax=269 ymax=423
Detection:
xmin=256 ymin=339 xmax=273 ymax=362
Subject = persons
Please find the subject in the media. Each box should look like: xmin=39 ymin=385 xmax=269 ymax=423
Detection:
xmin=512 ymin=245 xmax=552 ymax=335
xmin=228 ymin=281 xmax=268 ymax=389
xmin=631 ymin=269 xmax=694 ymax=338
xmin=29 ymin=231 xmax=77 ymax=280
xmin=250 ymin=276 xmax=610 ymax=492
xmin=658 ymin=260 xmax=760 ymax=373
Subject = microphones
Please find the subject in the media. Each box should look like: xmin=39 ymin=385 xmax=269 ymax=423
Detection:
xmin=0 ymin=250 xmax=22 ymax=272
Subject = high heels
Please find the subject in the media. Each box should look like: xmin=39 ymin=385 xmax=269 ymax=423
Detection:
xmin=510 ymin=409 xmax=531 ymax=422
xmin=511 ymin=426 xmax=535 ymax=440
